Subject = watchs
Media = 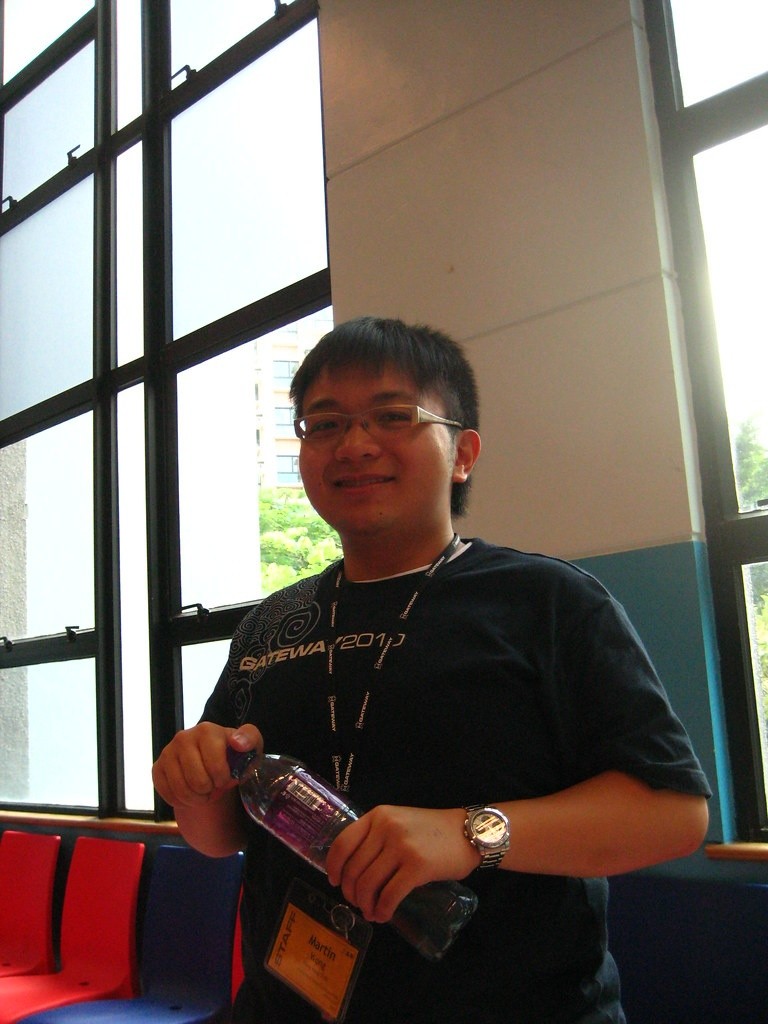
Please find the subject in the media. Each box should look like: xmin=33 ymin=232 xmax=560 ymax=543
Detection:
xmin=462 ymin=803 xmax=510 ymax=870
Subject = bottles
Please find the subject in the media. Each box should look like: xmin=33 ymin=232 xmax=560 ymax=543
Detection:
xmin=214 ymin=725 xmax=477 ymax=962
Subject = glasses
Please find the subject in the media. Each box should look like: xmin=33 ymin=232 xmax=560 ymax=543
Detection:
xmin=294 ymin=405 xmax=463 ymax=442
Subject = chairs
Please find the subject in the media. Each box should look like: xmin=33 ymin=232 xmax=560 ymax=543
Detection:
xmin=0 ymin=836 xmax=145 ymax=1024
xmin=0 ymin=830 xmax=63 ymax=979
xmin=605 ymin=874 xmax=768 ymax=1024
xmin=18 ymin=844 xmax=244 ymax=1024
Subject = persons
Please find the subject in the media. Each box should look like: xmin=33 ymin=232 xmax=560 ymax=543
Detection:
xmin=151 ymin=317 xmax=710 ymax=1024
